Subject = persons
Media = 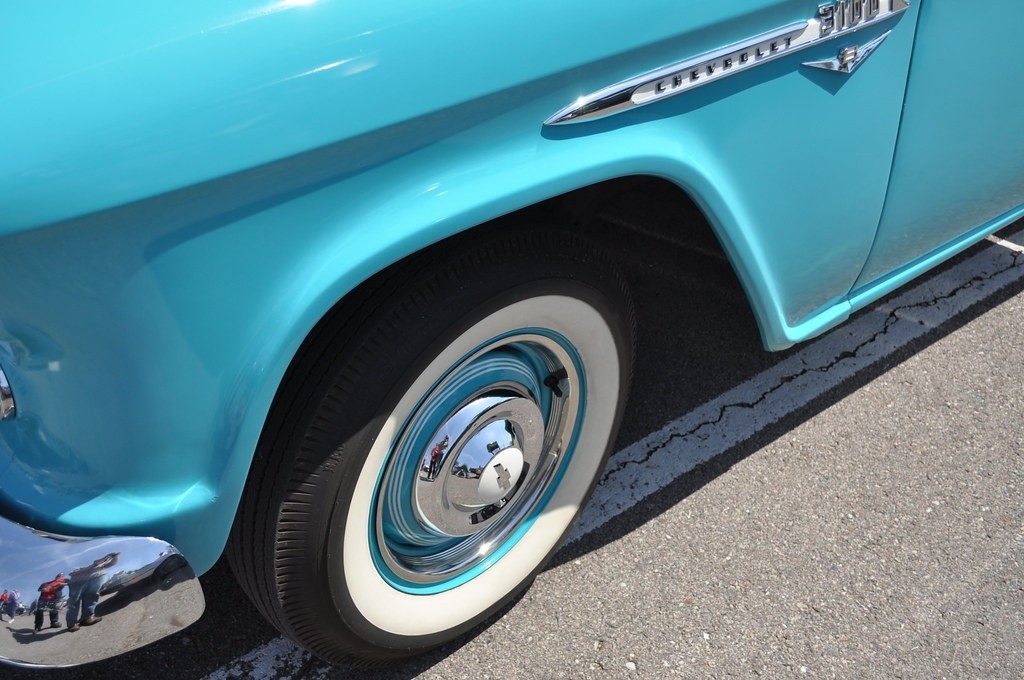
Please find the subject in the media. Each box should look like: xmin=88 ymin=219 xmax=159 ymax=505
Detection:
xmin=66 ymin=552 xmax=119 ymax=631
xmin=0 ymin=588 xmax=18 ymax=624
xmin=428 ymin=434 xmax=449 ymax=480
xmin=33 ymin=572 xmax=69 ymax=632
xmin=29 ymin=599 xmax=37 ymax=615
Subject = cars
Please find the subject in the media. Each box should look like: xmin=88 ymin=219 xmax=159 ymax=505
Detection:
xmin=98 ymin=545 xmax=184 ymax=598
xmin=2 ymin=0 xmax=1024 ymax=678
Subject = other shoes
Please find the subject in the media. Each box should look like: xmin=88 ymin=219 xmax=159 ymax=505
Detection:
xmin=51 ymin=622 xmax=63 ymax=627
xmin=432 ymin=475 xmax=434 ymax=479
xmin=8 ymin=618 xmax=15 ymax=623
xmin=428 ymin=477 xmax=430 ymax=479
xmin=80 ymin=616 xmax=101 ymax=625
xmin=68 ymin=624 xmax=79 ymax=632
xmin=34 ymin=626 xmax=41 ymax=633
